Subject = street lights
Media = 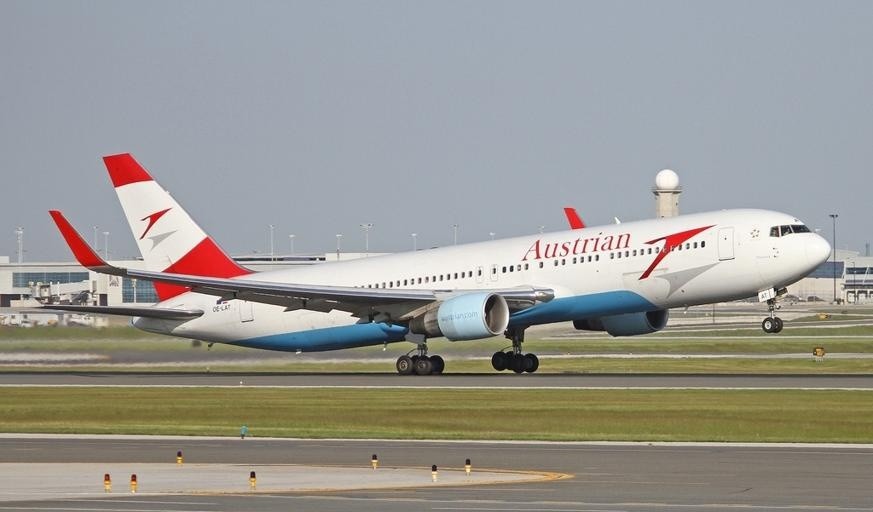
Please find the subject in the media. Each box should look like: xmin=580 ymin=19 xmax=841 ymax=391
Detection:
xmin=13 ymin=225 xmax=27 ymax=263
xmin=269 ymin=222 xmax=497 ymax=260
xmin=829 ymin=214 xmax=838 ymax=305
xmin=92 ymin=224 xmax=110 ymax=261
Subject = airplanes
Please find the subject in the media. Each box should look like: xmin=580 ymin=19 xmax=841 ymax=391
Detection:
xmin=1 ymin=151 xmax=835 ymax=375
